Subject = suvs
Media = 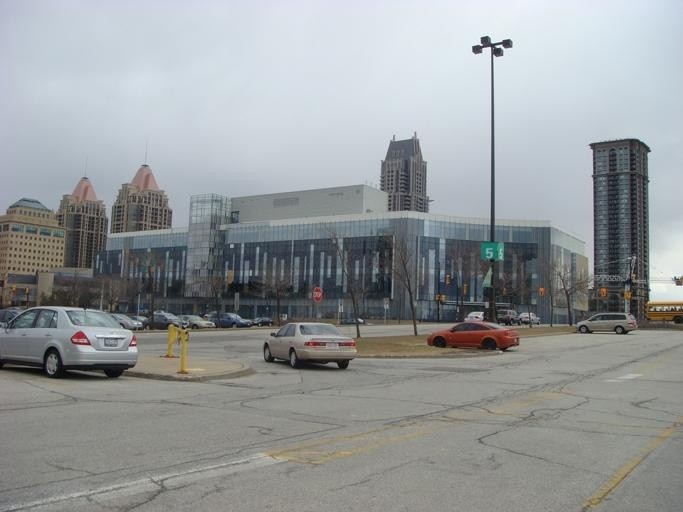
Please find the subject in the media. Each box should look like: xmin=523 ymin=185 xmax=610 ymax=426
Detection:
xmin=496 ymin=309 xmax=523 ymax=326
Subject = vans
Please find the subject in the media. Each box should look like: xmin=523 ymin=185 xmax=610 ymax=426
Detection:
xmin=572 ymin=310 xmax=640 ymax=336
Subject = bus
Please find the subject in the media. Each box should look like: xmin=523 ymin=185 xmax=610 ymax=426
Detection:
xmin=643 ymin=299 xmax=683 ymax=324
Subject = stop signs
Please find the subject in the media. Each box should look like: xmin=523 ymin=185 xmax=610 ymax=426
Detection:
xmin=313 ymin=288 xmax=322 ymax=302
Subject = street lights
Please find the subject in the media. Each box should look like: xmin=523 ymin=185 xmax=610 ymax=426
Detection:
xmin=471 ymin=33 xmax=515 ymax=329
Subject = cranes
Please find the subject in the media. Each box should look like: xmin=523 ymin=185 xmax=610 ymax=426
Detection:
xmin=584 ymin=255 xmax=682 ymax=313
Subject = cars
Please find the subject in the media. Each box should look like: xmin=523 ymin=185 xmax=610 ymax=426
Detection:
xmin=339 ymin=316 xmax=368 ymax=326
xmin=0 ymin=305 xmax=139 ymax=379
xmin=426 ymin=320 xmax=521 ymax=352
xmin=263 ymin=321 xmax=358 ymax=371
xmin=518 ymin=310 xmax=542 ymax=326
xmin=463 ymin=310 xmax=486 ymax=322
xmin=0 ymin=304 xmax=274 ymax=331
